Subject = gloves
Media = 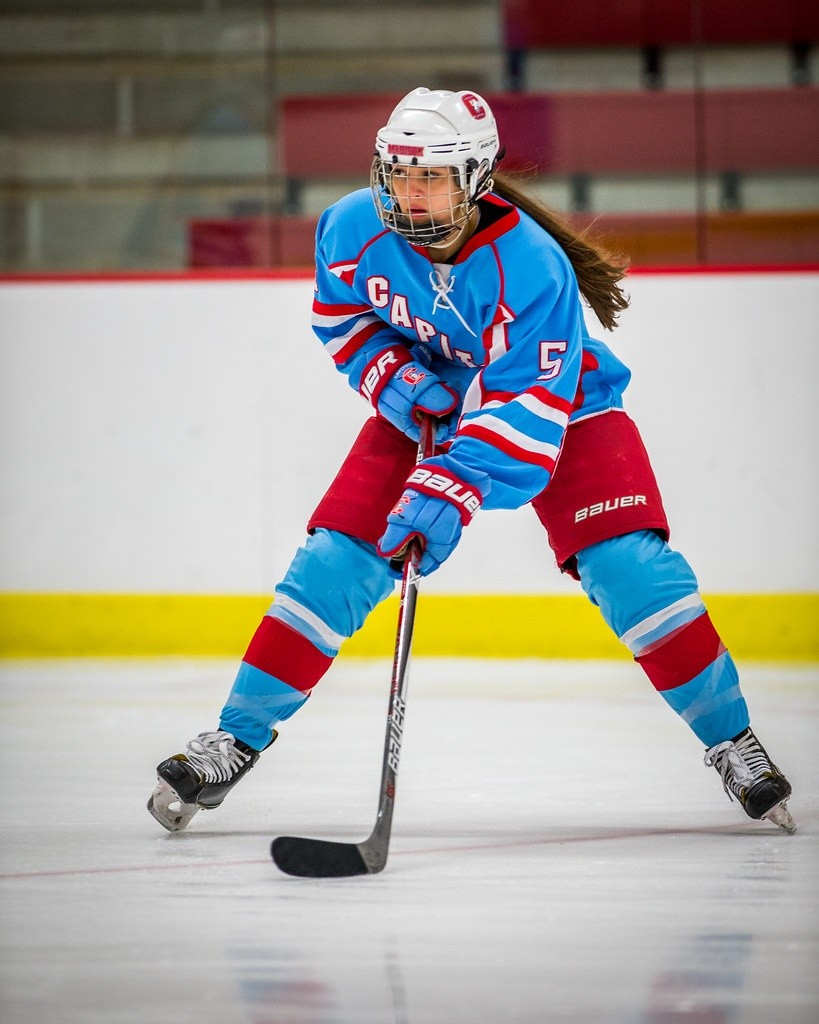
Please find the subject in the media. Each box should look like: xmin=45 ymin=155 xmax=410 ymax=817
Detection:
xmin=348 ymin=342 xmax=465 ymax=445
xmin=377 ymin=455 xmax=492 ymax=581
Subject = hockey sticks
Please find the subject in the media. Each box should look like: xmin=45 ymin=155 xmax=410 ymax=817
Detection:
xmin=265 ymin=413 xmax=439 ymax=879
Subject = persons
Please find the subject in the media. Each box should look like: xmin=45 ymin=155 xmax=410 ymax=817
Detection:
xmin=146 ymin=86 xmax=798 ymax=835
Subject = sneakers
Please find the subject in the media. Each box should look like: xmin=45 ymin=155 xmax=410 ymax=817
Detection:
xmin=146 ymin=727 xmax=278 ymax=832
xmin=703 ymin=725 xmax=796 ymax=833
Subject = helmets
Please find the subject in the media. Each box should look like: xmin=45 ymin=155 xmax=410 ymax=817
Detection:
xmin=369 ymin=87 xmax=499 ymax=248
